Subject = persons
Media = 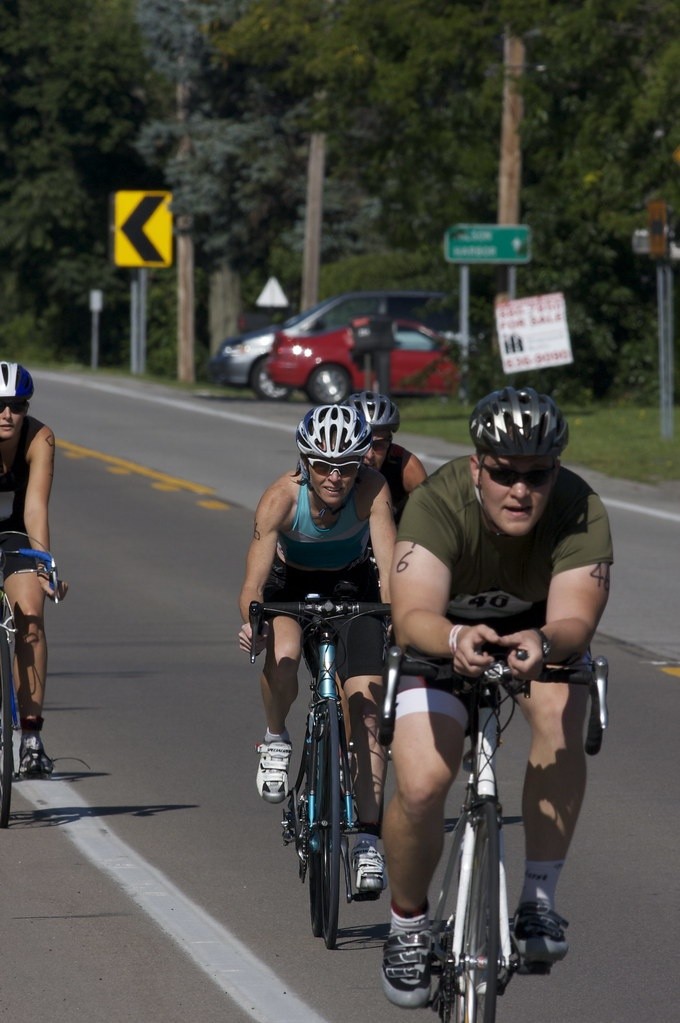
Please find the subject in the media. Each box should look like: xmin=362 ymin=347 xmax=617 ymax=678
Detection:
xmin=340 ymin=391 xmax=428 ymax=794
xmin=377 ymin=386 xmax=614 ymax=1010
xmin=0 ymin=361 xmax=69 ymax=773
xmin=238 ymin=403 xmax=397 ymax=893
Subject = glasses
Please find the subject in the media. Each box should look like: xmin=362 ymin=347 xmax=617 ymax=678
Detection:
xmin=308 ymin=457 xmax=361 ymax=478
xmin=480 ymin=462 xmax=557 ymax=487
xmin=0 ymin=402 xmax=27 ymax=415
xmin=372 ymin=440 xmax=393 ymax=453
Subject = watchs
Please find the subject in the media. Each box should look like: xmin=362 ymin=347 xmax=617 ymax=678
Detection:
xmin=535 ymin=628 xmax=551 ymax=663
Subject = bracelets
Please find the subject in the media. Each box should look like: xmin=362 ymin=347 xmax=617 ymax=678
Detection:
xmin=448 ymin=625 xmax=463 ymax=655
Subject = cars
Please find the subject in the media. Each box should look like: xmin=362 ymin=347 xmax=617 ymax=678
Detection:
xmin=216 ymin=289 xmax=451 ymax=400
xmin=265 ymin=314 xmax=460 ymax=406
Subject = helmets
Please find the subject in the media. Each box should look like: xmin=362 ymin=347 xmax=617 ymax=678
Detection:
xmin=296 ymin=404 xmax=373 ymax=461
xmin=470 ymin=386 xmax=571 ymax=459
xmin=342 ymin=392 xmax=402 ymax=432
xmin=0 ymin=360 xmax=34 ymax=400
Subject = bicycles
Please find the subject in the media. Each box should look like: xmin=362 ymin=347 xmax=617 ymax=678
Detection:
xmin=249 ymin=580 xmax=395 ymax=948
xmin=0 ymin=531 xmax=57 ymax=830
xmin=367 ymin=641 xmax=606 ymax=1023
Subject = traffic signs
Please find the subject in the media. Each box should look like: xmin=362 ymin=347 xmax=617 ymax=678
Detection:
xmin=444 ymin=223 xmax=531 ymax=265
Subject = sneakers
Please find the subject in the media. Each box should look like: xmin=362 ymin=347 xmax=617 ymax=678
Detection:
xmin=351 ymin=841 xmax=387 ymax=893
xmin=19 ymin=737 xmax=55 ymax=775
xmin=382 ymin=931 xmax=434 ymax=1009
xmin=257 ymin=740 xmax=295 ymax=804
xmin=514 ymin=904 xmax=569 ymax=961
